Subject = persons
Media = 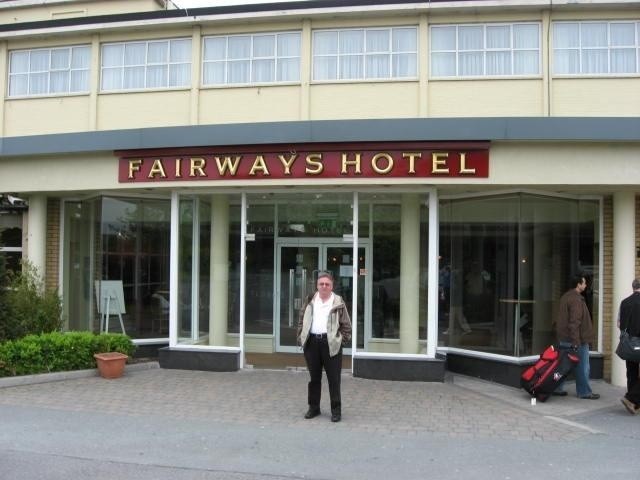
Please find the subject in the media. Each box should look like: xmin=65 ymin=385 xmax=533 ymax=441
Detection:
xmin=549 ymin=275 xmax=601 ymax=401
xmin=296 ymin=272 xmax=352 ymax=422
xmin=616 ymin=277 xmax=639 ymax=414
xmin=441 ymin=270 xmax=473 ymax=336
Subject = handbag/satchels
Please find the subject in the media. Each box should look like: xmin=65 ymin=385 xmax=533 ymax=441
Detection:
xmin=615 ymin=331 xmax=640 ymax=363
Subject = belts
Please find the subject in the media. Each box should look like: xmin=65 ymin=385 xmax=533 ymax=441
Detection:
xmin=310 ymin=333 xmax=327 ymax=340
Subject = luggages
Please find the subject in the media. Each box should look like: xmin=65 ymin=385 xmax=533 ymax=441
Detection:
xmin=519 ymin=344 xmax=579 ymax=403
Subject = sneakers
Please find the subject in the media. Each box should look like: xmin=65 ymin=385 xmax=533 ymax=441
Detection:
xmin=620 ymin=396 xmax=636 ymax=414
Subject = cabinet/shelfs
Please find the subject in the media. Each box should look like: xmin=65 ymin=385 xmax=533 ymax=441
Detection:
xmin=92 ymin=279 xmax=128 ymax=334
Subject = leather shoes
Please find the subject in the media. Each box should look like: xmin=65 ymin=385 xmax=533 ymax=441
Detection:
xmin=550 ymin=391 xmax=568 ymax=396
xmin=305 ymin=409 xmax=320 ymax=419
xmin=331 ymin=414 xmax=341 ymax=422
xmin=581 ymin=393 xmax=600 ymax=400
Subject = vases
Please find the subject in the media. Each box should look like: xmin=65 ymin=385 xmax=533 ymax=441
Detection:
xmin=94 ymin=351 xmax=126 ymax=378
